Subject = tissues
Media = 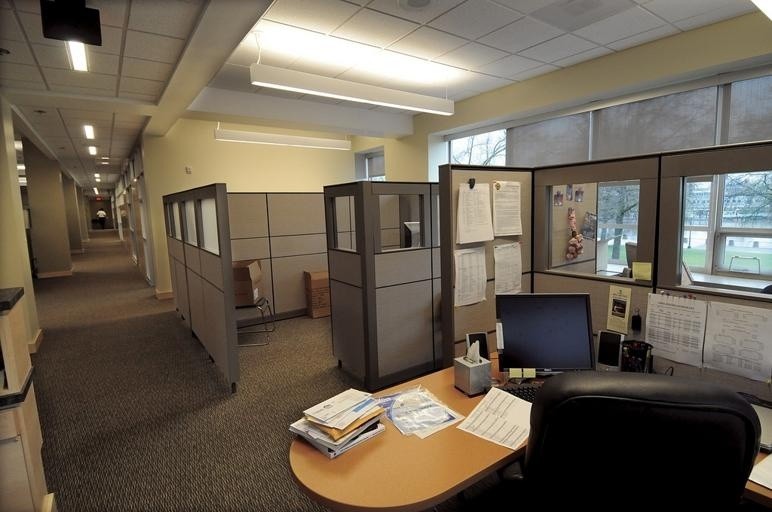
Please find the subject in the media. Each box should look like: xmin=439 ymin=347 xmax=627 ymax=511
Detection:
xmin=452 ymin=339 xmax=493 ymax=397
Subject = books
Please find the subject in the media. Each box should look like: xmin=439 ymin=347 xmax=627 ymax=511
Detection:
xmin=292 ymin=389 xmax=385 ymax=460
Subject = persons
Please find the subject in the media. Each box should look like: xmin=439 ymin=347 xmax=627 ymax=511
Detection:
xmin=97 ymin=208 xmax=106 ymax=230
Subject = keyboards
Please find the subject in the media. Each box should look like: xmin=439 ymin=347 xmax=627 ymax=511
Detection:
xmin=484 ymin=383 xmax=544 ymax=404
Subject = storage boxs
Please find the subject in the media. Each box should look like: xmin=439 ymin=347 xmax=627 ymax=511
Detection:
xmin=233 ymin=259 xmax=263 ymax=305
xmin=306 ymin=269 xmax=331 ymax=318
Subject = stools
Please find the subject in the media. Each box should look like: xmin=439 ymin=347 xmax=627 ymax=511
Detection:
xmin=235 ymin=298 xmax=276 ymax=346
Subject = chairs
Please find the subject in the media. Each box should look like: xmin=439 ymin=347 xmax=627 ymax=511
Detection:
xmin=494 ymin=370 xmax=763 ymax=509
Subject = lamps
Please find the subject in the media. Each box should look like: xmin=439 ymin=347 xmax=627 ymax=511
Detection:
xmin=214 ymin=121 xmax=351 ymax=150
xmin=249 ymin=31 xmax=455 ymax=117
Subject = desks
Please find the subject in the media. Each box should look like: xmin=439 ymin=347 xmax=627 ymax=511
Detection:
xmin=289 ymin=353 xmax=772 ymax=510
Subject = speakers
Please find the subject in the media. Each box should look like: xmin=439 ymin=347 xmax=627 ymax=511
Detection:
xmin=595 ymin=330 xmax=626 ymax=371
xmin=465 ymin=332 xmax=490 ymax=361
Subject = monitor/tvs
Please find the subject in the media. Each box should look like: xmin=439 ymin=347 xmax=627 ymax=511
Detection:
xmin=495 ymin=293 xmax=596 ymax=384
